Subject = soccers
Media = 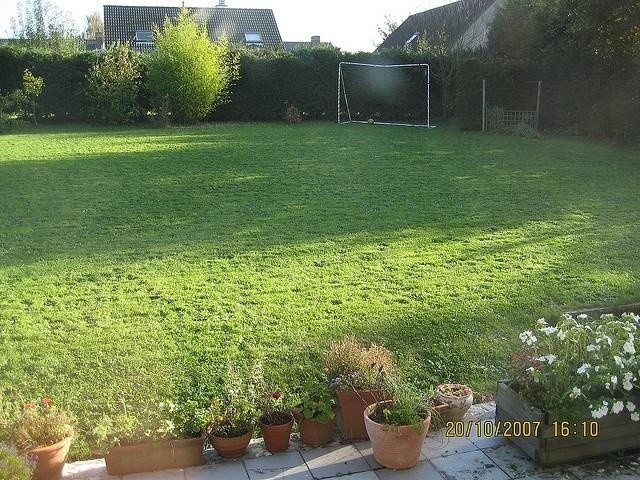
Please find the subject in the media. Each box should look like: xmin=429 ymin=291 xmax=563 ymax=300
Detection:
xmin=368 ymin=118 xmax=374 ymax=124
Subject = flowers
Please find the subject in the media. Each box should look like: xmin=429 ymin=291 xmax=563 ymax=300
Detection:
xmin=509 ymin=311 xmax=640 ymax=423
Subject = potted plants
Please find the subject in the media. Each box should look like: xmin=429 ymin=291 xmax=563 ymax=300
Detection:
xmin=290 ymin=374 xmax=336 ymax=448
xmin=94 ymin=397 xmax=213 ymax=475
xmin=334 ymin=370 xmax=395 ymax=442
xmin=255 ymin=392 xmax=294 ymax=452
xmin=340 ymin=365 xmax=443 ymax=470
xmin=206 ymin=391 xmax=253 ymax=459
xmin=5 ymin=409 xmax=74 ymax=480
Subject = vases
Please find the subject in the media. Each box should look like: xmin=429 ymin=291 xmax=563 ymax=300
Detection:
xmin=494 ymin=379 xmax=640 ymax=466
xmin=435 ymin=383 xmax=473 ymax=420
